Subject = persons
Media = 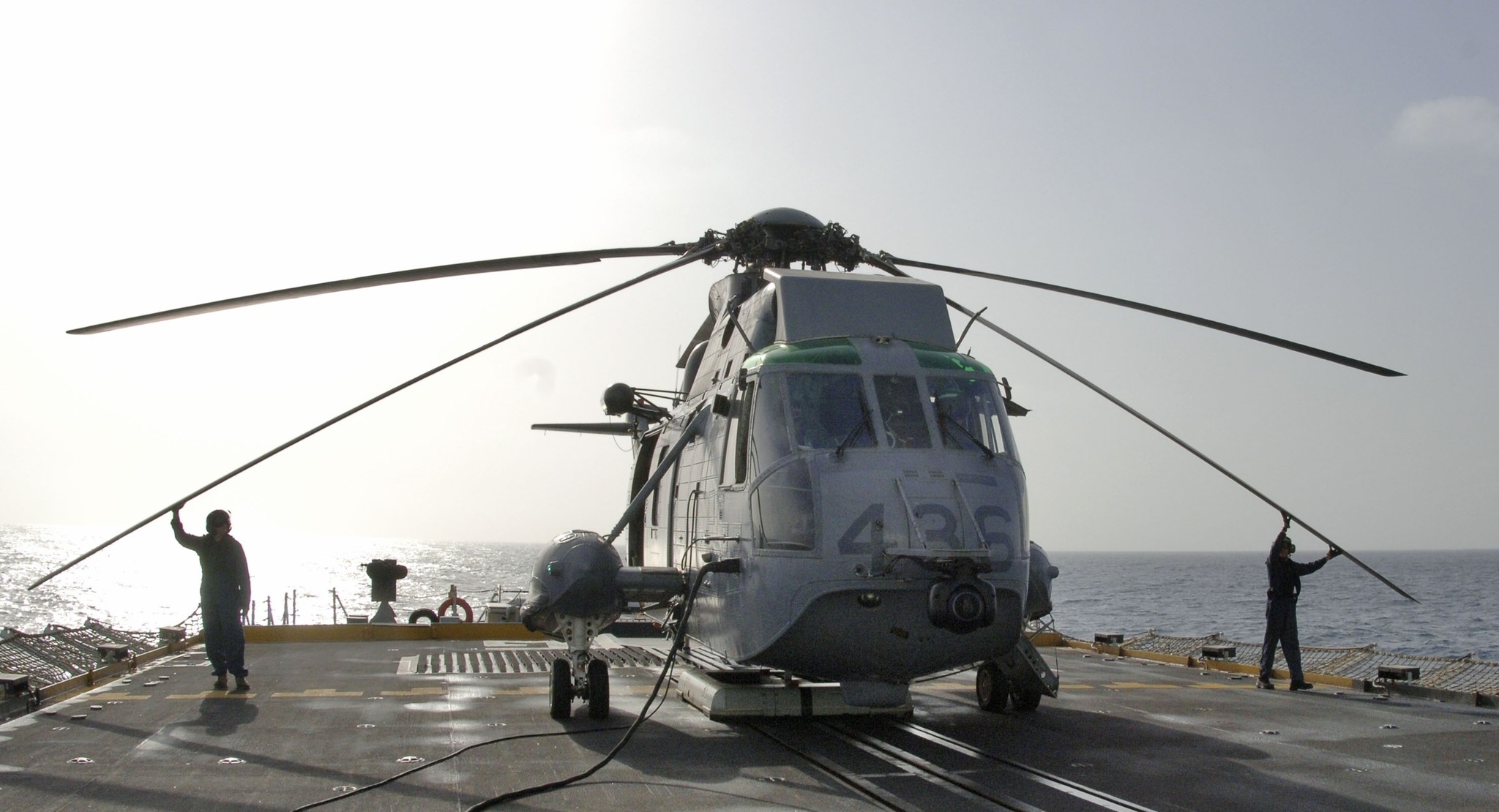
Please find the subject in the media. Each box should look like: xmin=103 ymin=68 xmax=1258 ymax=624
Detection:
xmin=170 ymin=502 xmax=251 ymax=690
xmin=779 ymin=390 xmax=979 ymax=451
xmin=1256 ymin=512 xmax=1342 ymax=690
xmin=631 ymin=512 xmax=645 ymax=567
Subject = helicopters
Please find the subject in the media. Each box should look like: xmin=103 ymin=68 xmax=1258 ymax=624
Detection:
xmin=27 ymin=207 xmax=1423 ymax=726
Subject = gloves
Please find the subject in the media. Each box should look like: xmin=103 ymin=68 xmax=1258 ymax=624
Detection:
xmin=1327 ymin=545 xmax=1342 ymax=560
xmin=1281 ymin=511 xmax=1292 ymax=529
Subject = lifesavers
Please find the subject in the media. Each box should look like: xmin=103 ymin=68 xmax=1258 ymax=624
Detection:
xmin=437 ymin=597 xmax=474 ymax=624
xmin=408 ymin=608 xmax=438 ymax=624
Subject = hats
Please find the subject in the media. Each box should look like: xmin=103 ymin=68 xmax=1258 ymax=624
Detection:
xmin=206 ymin=508 xmax=231 ymax=529
xmin=1279 ymin=537 xmax=1292 ymax=543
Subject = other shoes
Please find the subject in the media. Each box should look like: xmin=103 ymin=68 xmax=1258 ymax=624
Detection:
xmin=1289 ymin=681 xmax=1313 ymax=691
xmin=214 ymin=676 xmax=229 ymax=691
xmin=1256 ymin=677 xmax=1273 ymax=688
xmin=234 ymin=675 xmax=251 ymax=690
